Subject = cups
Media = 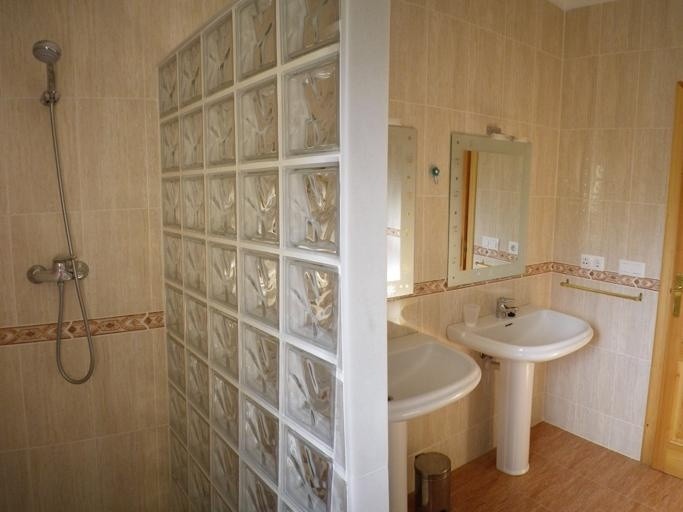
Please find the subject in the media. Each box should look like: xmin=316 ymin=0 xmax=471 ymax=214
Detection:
xmin=463 ymin=304 xmax=480 ymax=328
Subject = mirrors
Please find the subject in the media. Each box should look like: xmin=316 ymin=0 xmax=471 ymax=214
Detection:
xmin=448 ymin=131 xmax=533 ymax=288
xmin=388 ymin=126 xmax=417 ymax=301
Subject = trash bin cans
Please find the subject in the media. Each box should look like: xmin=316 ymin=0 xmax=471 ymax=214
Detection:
xmin=413 ymin=452 xmax=451 ymax=512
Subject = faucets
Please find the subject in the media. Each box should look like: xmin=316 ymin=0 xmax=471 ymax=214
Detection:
xmin=497 ymin=296 xmax=518 ymax=320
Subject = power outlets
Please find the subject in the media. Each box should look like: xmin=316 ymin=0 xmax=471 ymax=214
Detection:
xmin=580 ymin=254 xmax=604 ymax=271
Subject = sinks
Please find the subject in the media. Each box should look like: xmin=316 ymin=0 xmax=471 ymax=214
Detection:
xmin=445 ymin=307 xmax=594 ymax=361
xmin=388 ymin=331 xmax=475 ymax=419
